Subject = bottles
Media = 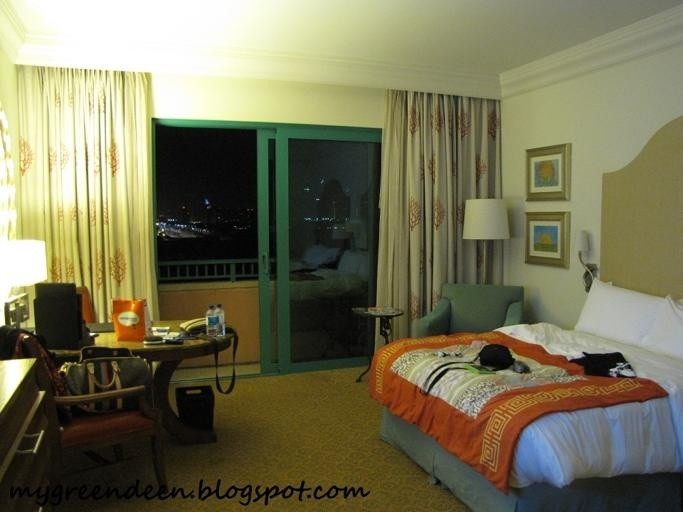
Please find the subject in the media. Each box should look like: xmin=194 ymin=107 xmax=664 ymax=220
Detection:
xmin=206 ymin=303 xmax=217 ymax=337
xmin=216 ymin=303 xmax=225 ymax=336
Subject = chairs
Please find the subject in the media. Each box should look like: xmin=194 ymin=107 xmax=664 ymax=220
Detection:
xmin=410 ymin=285 xmax=525 ymax=337
xmin=76 ymin=286 xmax=96 ymax=323
xmin=0 ymin=325 xmax=169 ymax=492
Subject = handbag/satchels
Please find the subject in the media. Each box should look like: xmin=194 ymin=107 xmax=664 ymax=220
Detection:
xmin=179 ymin=317 xmax=207 ymax=335
xmin=176 ymin=385 xmax=215 ymax=428
xmin=480 ymin=344 xmax=514 ymax=372
xmin=111 ymin=298 xmax=153 ymax=342
xmin=60 ymin=346 xmax=146 ymax=415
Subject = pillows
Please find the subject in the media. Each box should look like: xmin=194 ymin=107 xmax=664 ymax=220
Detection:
xmin=304 ymin=243 xmax=374 ymax=277
xmin=572 ymin=277 xmax=668 ymax=346
xmin=640 ymin=292 xmax=683 ymax=359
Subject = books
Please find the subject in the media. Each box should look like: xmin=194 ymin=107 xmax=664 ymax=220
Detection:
xmin=464 ymin=363 xmax=496 ymax=375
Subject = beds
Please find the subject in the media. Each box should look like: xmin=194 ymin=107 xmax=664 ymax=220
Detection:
xmin=291 ymin=250 xmax=374 ymax=333
xmin=369 ymin=116 xmax=682 ymax=512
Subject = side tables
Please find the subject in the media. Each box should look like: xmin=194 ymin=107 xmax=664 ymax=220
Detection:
xmin=352 ymin=307 xmax=403 ymax=383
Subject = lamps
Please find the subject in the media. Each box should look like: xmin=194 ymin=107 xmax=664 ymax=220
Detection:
xmin=332 ymin=223 xmax=357 ymax=249
xmin=575 ymin=228 xmax=596 ymax=293
xmin=462 ymin=198 xmax=510 ymax=284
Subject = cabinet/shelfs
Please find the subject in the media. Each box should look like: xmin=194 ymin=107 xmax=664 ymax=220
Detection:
xmin=0 ymin=356 xmax=57 ymax=512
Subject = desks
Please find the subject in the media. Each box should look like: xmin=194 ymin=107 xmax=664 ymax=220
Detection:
xmin=93 ymin=321 xmax=233 ymax=442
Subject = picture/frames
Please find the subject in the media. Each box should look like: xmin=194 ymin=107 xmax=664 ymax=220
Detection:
xmin=525 ymin=143 xmax=572 ymax=201
xmin=525 ymin=211 xmax=571 ymax=268
